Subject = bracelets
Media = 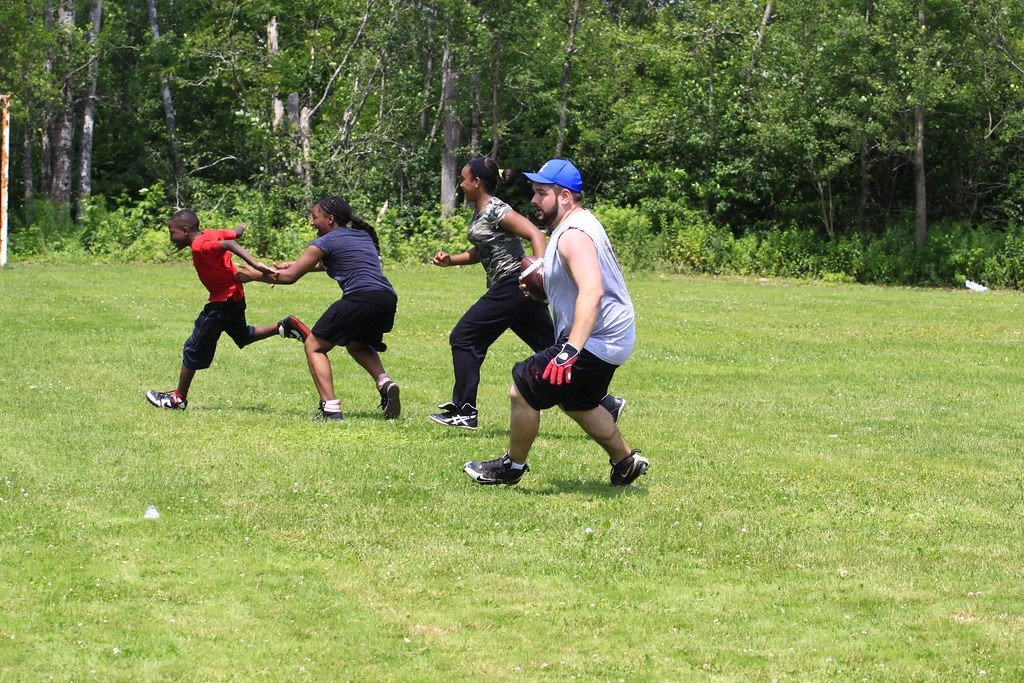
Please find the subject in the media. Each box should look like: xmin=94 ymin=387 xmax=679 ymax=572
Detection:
xmin=282 ymin=261 xmax=289 ymax=269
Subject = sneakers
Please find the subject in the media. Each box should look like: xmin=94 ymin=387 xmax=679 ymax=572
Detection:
xmin=315 ymin=399 xmax=343 ymax=422
xmin=608 ymin=449 xmax=649 ymax=486
xmin=464 ymin=456 xmax=531 ymax=486
xmin=278 ymin=315 xmax=312 ymax=346
xmin=599 ymin=391 xmax=626 ymax=426
xmin=375 ymin=380 xmax=403 ymax=422
xmin=145 ymin=388 xmax=187 ymax=410
xmin=431 ymin=401 xmax=479 ymax=432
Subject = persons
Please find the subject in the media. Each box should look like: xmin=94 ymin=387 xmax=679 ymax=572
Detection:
xmin=233 ymin=194 xmax=400 ymax=422
xmin=461 ymin=159 xmax=652 ymax=487
xmin=429 ymin=152 xmax=628 ymax=430
xmin=146 ymin=209 xmax=312 ymax=414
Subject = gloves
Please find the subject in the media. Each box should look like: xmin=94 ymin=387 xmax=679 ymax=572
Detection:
xmin=536 ymin=342 xmax=578 ymax=385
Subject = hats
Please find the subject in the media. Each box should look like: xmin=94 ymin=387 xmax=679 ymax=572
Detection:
xmin=522 ymin=157 xmax=586 ymax=197
